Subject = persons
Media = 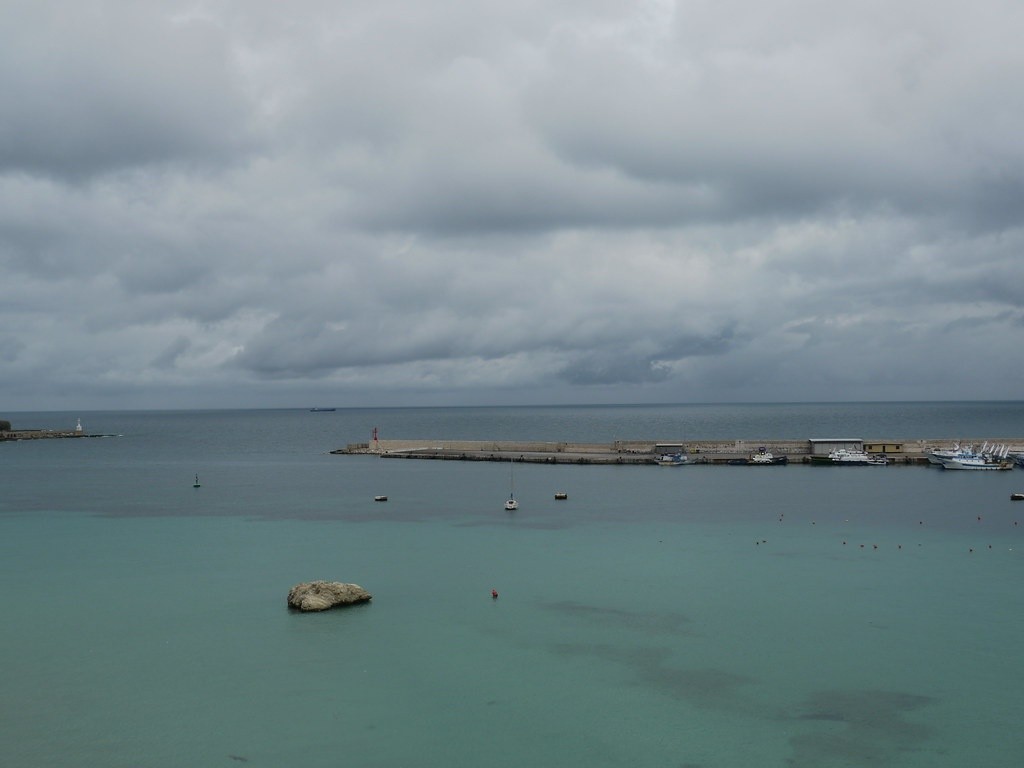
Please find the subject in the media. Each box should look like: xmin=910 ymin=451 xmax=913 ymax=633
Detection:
xmin=491 ymin=589 xmax=498 ymax=598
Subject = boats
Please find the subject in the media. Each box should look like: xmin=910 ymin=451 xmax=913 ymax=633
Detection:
xmin=505 ymin=491 xmax=519 ymax=510
xmin=747 ymin=447 xmax=790 ymax=466
xmin=828 ymin=442 xmax=890 ymax=466
xmin=654 ymin=449 xmax=698 ymax=466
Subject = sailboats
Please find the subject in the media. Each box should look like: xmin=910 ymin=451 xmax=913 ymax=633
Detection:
xmin=924 ymin=439 xmax=1015 ymax=471
